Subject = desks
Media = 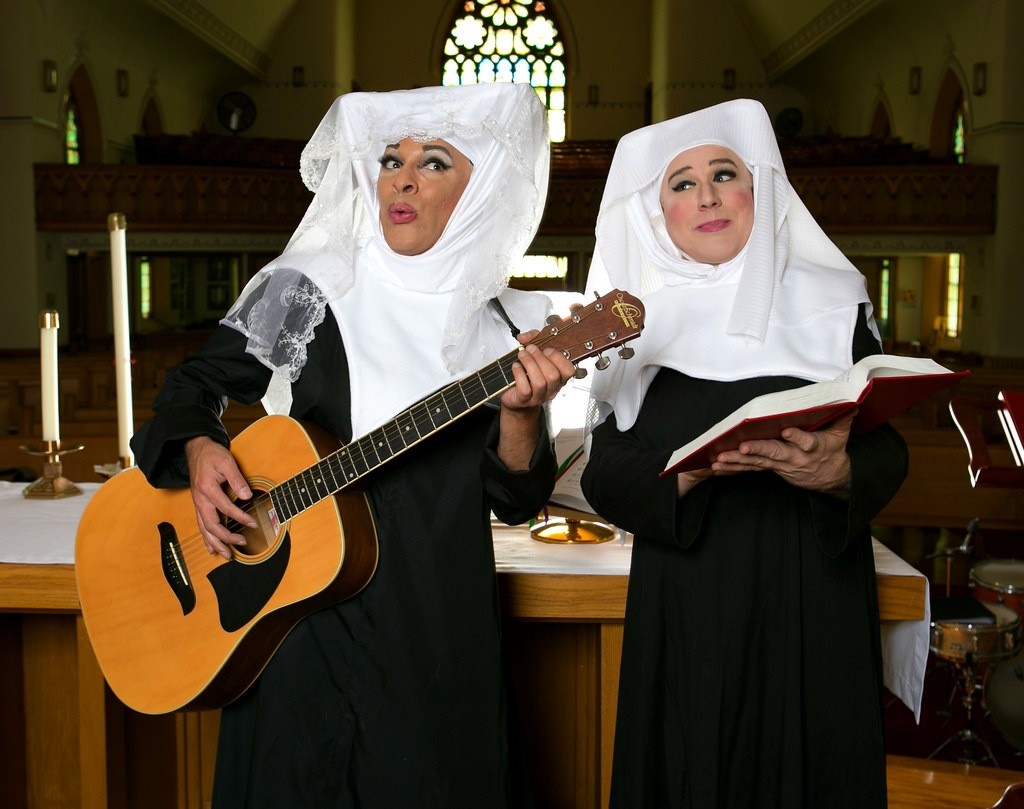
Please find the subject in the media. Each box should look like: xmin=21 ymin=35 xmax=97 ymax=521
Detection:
xmin=0 ymin=477 xmax=927 ymax=809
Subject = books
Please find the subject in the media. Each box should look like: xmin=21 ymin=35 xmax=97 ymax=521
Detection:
xmin=552 ymin=427 xmax=597 ymax=516
xmin=659 ymin=353 xmax=970 ymax=475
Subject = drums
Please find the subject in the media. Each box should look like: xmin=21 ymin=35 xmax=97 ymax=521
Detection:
xmin=930 ymin=602 xmax=1021 ymax=661
xmin=982 ymin=655 xmax=1024 ymax=752
xmin=970 ymin=561 xmax=1024 ymax=619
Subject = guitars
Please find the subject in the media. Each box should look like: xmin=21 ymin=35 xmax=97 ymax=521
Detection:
xmin=77 ymin=289 xmax=646 ymax=716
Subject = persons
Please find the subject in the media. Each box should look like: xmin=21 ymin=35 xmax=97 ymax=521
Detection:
xmin=129 ymin=82 xmax=577 ymax=809
xmin=579 ymin=99 xmax=908 ymax=808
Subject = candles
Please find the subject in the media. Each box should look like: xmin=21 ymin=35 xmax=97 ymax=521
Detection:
xmin=108 ymin=212 xmax=136 ymax=460
xmin=38 ymin=310 xmax=60 ymax=441
